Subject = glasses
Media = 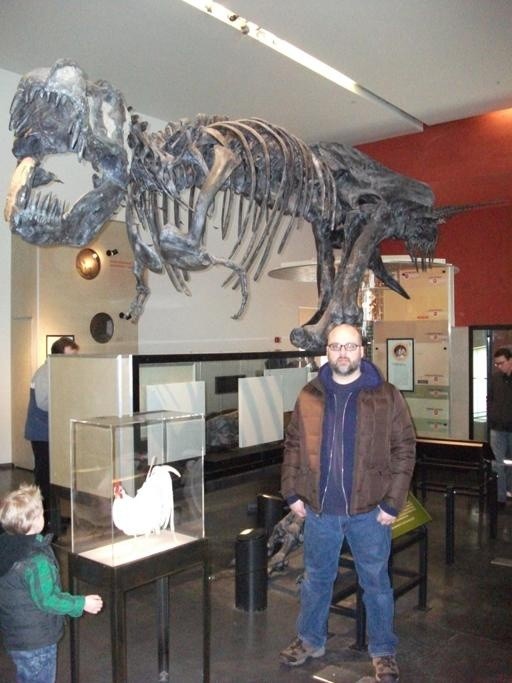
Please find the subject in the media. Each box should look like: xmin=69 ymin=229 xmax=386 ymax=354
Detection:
xmin=327 ymin=343 xmax=361 ymax=352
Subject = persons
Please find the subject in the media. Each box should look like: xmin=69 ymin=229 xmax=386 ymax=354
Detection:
xmin=1 ymin=483 xmax=105 ymax=682
xmin=486 ymin=348 xmax=510 ymax=512
xmin=24 ymin=337 xmax=80 ymax=538
xmin=280 ymin=322 xmax=418 ymax=682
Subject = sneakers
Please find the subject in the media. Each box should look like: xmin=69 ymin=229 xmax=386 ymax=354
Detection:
xmin=278 ymin=636 xmax=325 ymax=666
xmin=372 ymin=656 xmax=400 ymax=683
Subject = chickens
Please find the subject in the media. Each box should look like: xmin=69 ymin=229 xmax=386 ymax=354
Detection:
xmin=112 ymin=455 xmax=181 ymax=538
